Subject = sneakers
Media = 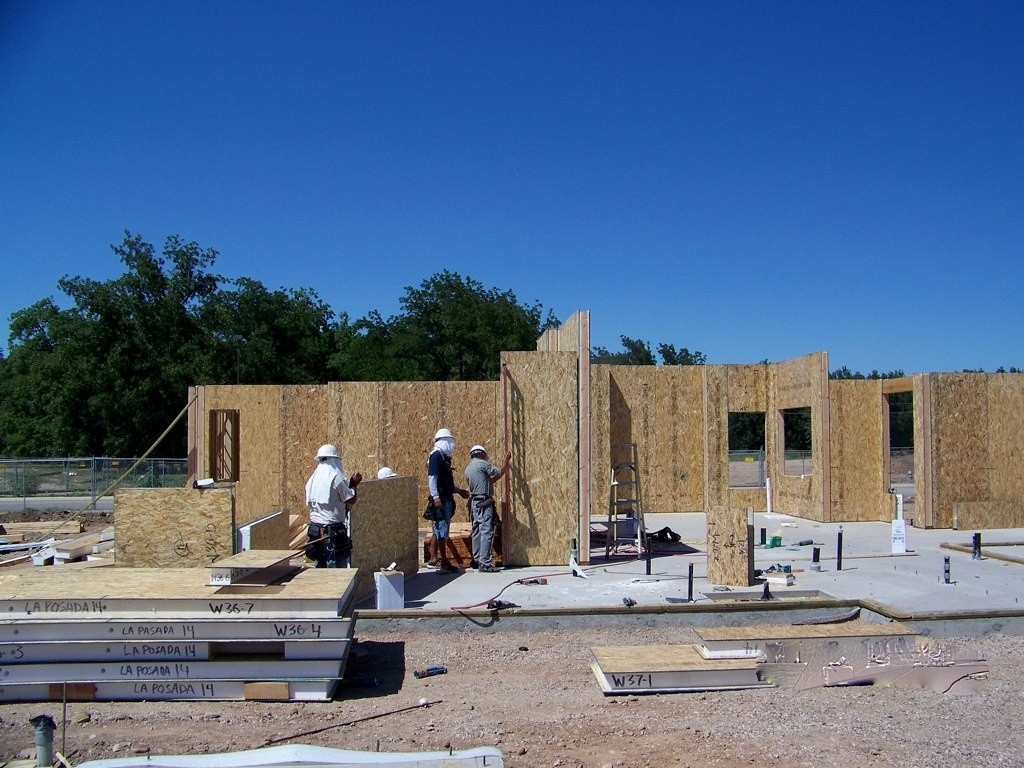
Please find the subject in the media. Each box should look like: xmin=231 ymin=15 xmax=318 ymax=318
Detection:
xmin=427 ymin=559 xmax=441 ymax=569
xmin=440 ymin=561 xmax=458 ymax=573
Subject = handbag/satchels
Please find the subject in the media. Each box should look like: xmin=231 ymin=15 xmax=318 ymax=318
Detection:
xmin=423 ymin=499 xmax=445 ymax=521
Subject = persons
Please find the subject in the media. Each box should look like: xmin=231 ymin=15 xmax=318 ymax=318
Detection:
xmin=465 ymin=444 xmax=512 ymax=573
xmin=304 ymin=444 xmax=362 ymax=569
xmin=422 ymin=428 xmax=470 ymax=572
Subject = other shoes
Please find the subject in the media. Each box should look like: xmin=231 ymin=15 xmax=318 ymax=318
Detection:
xmin=478 ymin=564 xmax=505 ymax=571
xmin=472 ymin=562 xmax=479 ymax=569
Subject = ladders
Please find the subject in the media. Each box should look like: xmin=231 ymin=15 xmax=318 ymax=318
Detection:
xmin=604 ymin=443 xmax=648 ymax=561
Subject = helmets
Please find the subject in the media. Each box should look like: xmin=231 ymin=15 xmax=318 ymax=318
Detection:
xmin=434 ymin=429 xmax=453 ymax=442
xmin=314 ymin=445 xmax=342 ymax=462
xmin=378 ymin=467 xmax=397 ymax=480
xmin=470 ymin=445 xmax=487 ymax=455
